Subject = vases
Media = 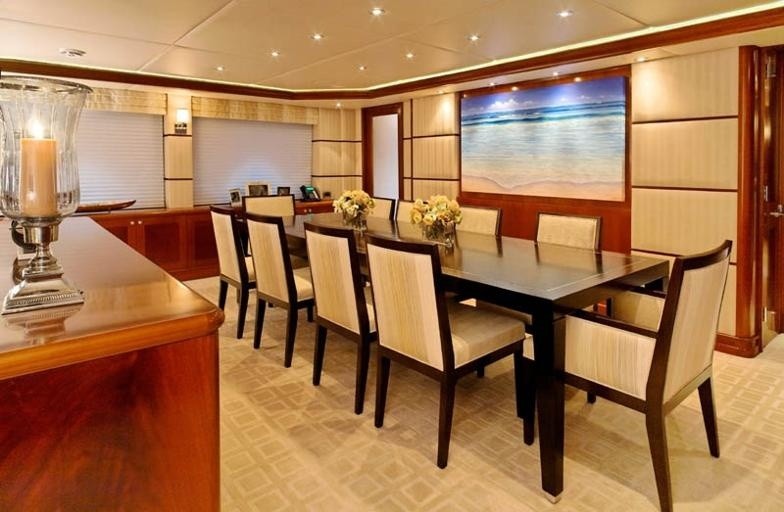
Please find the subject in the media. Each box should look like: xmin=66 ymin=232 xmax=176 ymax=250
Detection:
xmin=421 ymin=223 xmax=454 ymax=248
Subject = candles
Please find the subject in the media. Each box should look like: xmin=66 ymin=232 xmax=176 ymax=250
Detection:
xmin=21 ymin=117 xmax=57 ymax=215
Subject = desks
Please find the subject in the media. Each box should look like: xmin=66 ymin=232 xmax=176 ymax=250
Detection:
xmin=282 ymin=213 xmax=670 ymax=504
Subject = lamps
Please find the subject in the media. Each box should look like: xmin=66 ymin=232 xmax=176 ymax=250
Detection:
xmin=175 ymin=108 xmax=189 ymax=133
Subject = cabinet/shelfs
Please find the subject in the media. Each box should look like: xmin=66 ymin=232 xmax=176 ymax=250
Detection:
xmin=187 ymin=207 xmax=243 ymax=280
xmin=96 ymin=214 xmax=187 ymax=281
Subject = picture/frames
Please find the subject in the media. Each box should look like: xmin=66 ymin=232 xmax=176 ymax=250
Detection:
xmin=246 ymin=182 xmax=271 ymax=195
xmin=278 ymin=187 xmax=290 ymax=195
xmin=230 ymin=189 xmax=241 ymax=206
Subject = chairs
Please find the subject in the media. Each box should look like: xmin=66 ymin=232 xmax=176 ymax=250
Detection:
xmin=303 ymin=222 xmax=377 ymax=414
xmin=363 ymin=231 xmax=533 ymax=469
xmin=444 ymin=206 xmax=502 ymax=303
xmin=368 ymin=197 xmax=396 ymax=221
xmin=244 ymin=211 xmax=314 ymax=367
xmin=395 ymin=200 xmax=428 ymax=223
xmin=242 ymin=194 xmax=308 ymax=269
xmin=476 ymin=211 xmax=602 ymax=327
xmin=564 ymin=240 xmax=732 ymax=512
xmin=210 ymin=205 xmax=308 ymax=339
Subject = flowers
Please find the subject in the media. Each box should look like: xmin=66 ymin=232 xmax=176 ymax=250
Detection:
xmin=332 ymin=190 xmax=376 ymax=228
xmin=410 ymin=194 xmax=462 ymax=240
